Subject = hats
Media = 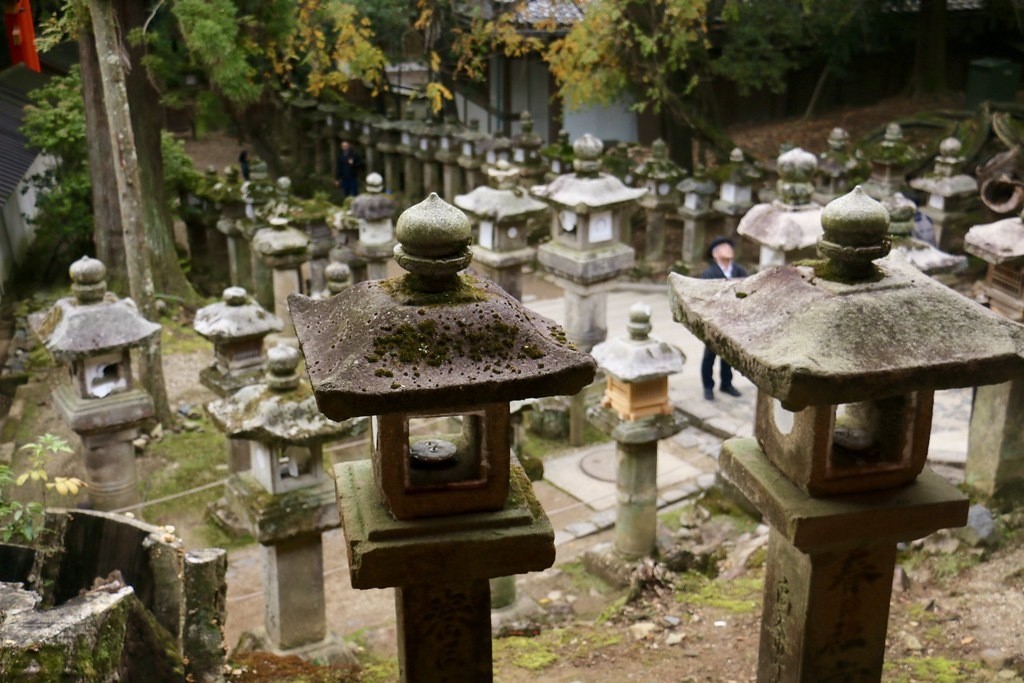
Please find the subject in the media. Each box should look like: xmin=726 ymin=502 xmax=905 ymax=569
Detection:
xmin=702 ymin=236 xmax=735 ymax=259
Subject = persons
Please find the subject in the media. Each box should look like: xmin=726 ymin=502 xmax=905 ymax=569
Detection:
xmin=699 ymin=237 xmax=747 ymax=400
xmin=909 ymin=196 xmax=937 ymax=277
xmin=239 ymin=149 xmax=252 ymax=180
xmin=334 ymin=141 xmax=365 ymax=199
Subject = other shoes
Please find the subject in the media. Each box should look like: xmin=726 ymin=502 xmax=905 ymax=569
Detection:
xmin=721 ymin=384 xmax=741 ymax=397
xmin=704 ymin=388 xmax=713 ymax=400
